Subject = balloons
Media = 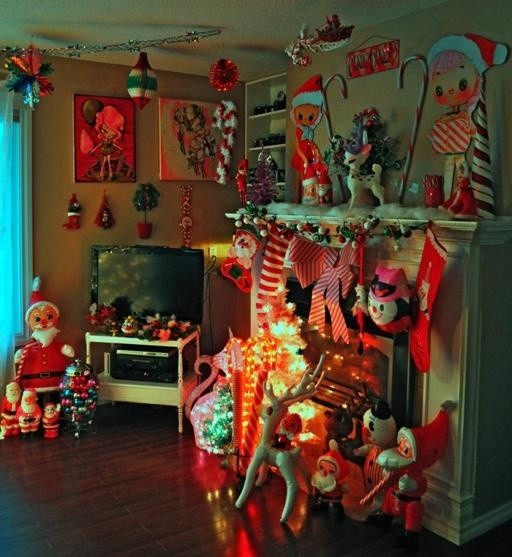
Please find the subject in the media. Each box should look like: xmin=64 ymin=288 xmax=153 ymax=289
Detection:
xmin=367 ymin=264 xmax=414 ymax=333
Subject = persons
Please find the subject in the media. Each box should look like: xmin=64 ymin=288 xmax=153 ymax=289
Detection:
xmin=311 ymin=398 xmax=458 ymax=536
xmin=103 ymin=208 xmax=112 ymax=228
xmin=237 ymin=161 xmax=333 ymax=206
xmin=67 ymin=201 xmax=83 ymax=216
xmin=0 ymin=277 xmax=75 ymax=441
xmin=225 ymin=225 xmax=262 ymax=279
xmin=272 ymin=413 xmax=302 ymax=449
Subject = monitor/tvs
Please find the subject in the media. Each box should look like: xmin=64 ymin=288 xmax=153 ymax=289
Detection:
xmin=88 ymin=246 xmax=205 ymax=325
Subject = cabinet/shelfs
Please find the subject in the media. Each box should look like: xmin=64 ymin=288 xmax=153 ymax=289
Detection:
xmin=80 ymin=327 xmax=204 ymax=434
xmin=242 ymin=68 xmax=299 ymax=204
